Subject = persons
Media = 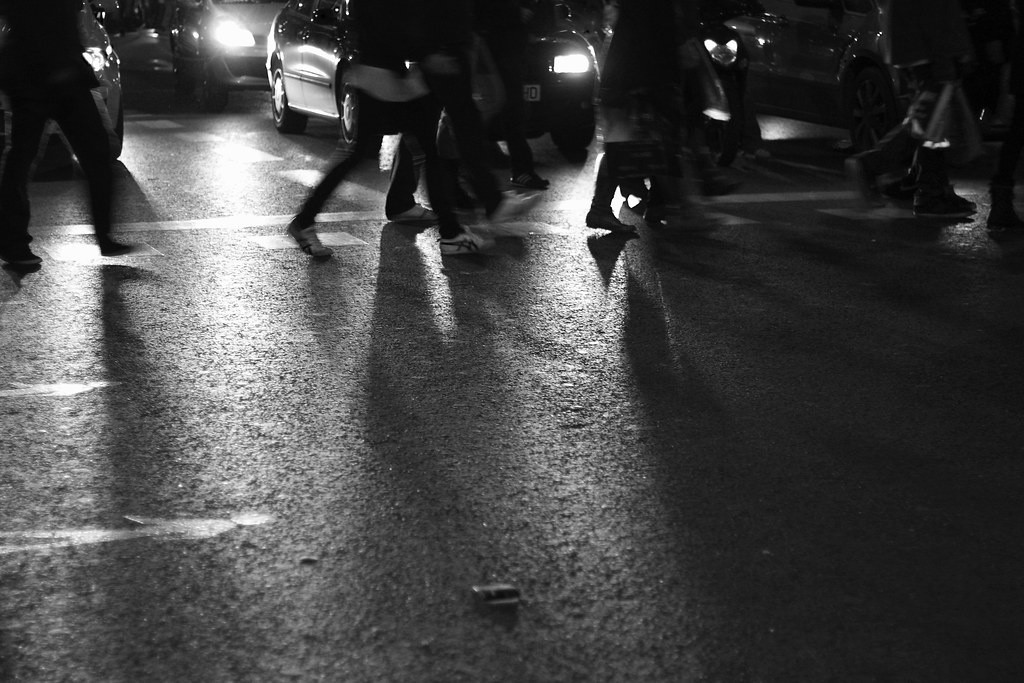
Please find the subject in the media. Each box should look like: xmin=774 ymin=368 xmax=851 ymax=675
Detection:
xmin=286 ymin=2 xmax=498 ymax=263
xmin=0 ymin=0 xmax=135 ymax=263
xmin=845 ymin=0 xmax=954 ymax=201
xmin=385 ymin=2 xmax=484 ymax=222
xmin=449 ymin=2 xmax=552 ymax=195
xmin=578 ymin=1 xmax=683 ymax=237
xmin=912 ymin=1 xmax=1024 ymax=231
xmin=619 ymin=0 xmax=743 ymax=198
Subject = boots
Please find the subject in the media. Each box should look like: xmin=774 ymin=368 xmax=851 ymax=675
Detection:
xmin=912 ymin=140 xmax=975 ymax=219
xmin=847 ymin=116 xmax=920 ymax=209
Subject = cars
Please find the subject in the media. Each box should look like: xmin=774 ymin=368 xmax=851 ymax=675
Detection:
xmin=0 ymin=1 xmax=124 ymax=178
xmin=263 ymin=3 xmax=600 ymax=158
xmin=582 ymin=6 xmax=1022 ymax=182
xmin=170 ymin=0 xmax=284 ymax=108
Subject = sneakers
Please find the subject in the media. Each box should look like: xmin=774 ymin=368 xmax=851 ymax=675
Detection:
xmin=510 ymin=168 xmax=548 ymax=191
xmin=284 ymin=219 xmax=331 ymax=260
xmin=440 ymin=225 xmax=498 ymax=258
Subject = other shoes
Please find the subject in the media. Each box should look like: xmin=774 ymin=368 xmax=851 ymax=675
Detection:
xmin=0 ymin=232 xmax=46 ymax=268
xmin=983 ymin=204 xmax=1022 ymax=234
xmin=583 ymin=208 xmax=639 ymax=234
xmin=392 ymin=204 xmax=437 ymax=224
